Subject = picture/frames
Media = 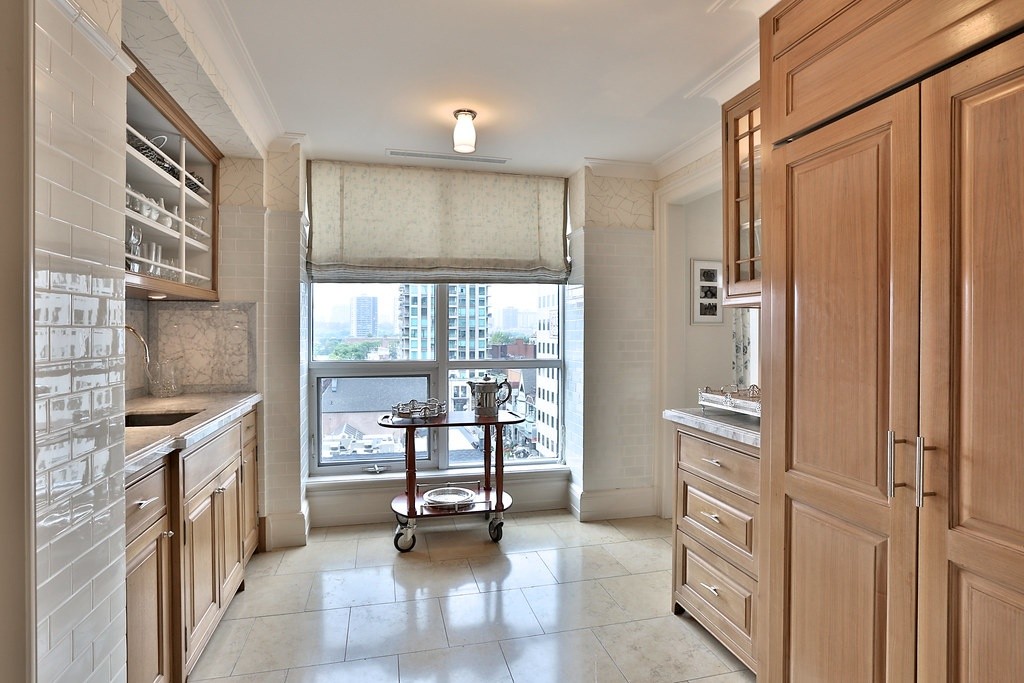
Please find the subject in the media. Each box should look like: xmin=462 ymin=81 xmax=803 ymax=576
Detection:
xmin=690 ymin=257 xmax=725 ymax=326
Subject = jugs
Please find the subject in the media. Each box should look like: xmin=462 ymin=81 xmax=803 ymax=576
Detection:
xmin=466 ymin=373 xmax=512 ymax=417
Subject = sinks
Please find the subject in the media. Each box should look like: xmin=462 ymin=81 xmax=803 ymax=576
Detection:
xmin=125 ymin=408 xmax=207 ymax=428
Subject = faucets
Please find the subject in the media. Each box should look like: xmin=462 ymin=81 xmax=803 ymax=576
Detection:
xmin=125 ymin=324 xmax=150 ymax=364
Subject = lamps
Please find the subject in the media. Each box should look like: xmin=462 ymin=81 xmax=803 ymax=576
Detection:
xmin=453 ymin=108 xmax=478 ymax=153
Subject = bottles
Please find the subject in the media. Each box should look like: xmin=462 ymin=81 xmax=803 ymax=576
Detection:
xmin=125 ymin=183 xmax=205 ymax=286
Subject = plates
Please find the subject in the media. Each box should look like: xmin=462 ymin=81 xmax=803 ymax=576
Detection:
xmin=423 ymin=486 xmax=476 ymax=509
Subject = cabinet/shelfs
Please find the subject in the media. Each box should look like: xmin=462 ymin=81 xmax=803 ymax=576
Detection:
xmin=376 ymin=408 xmax=526 ymax=551
xmin=124 ymin=391 xmax=264 ymax=683
xmin=758 ymin=0 xmax=1024 ymax=683
xmin=665 ymin=408 xmax=761 ymax=676
xmin=720 ymin=79 xmax=761 ymax=307
xmin=121 ymin=42 xmax=224 ymax=303
